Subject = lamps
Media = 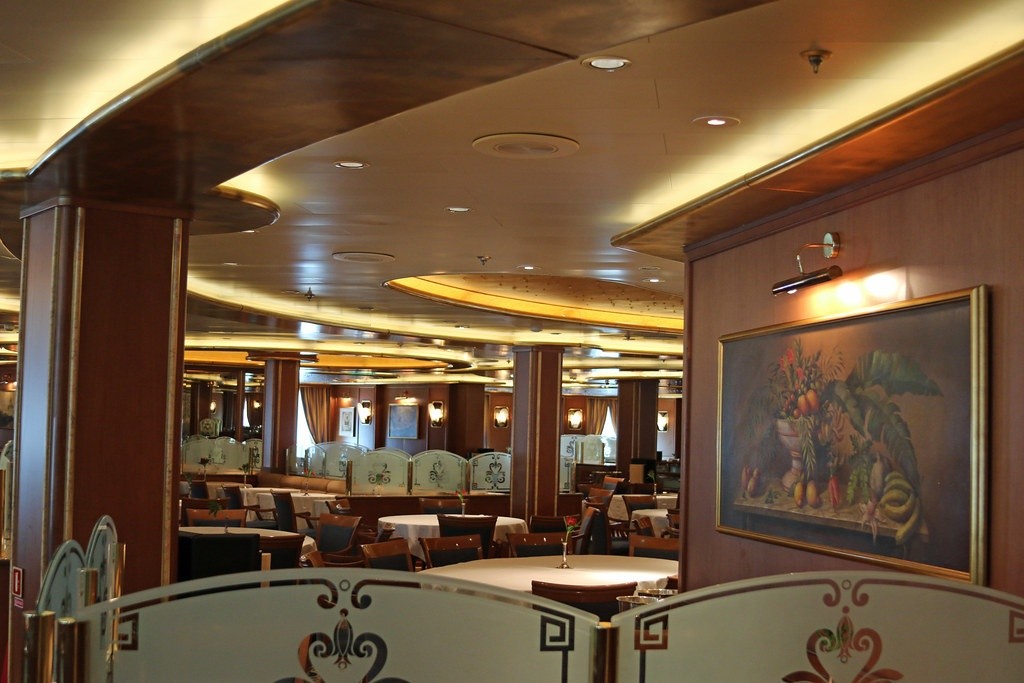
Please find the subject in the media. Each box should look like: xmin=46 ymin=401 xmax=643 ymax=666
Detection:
xmin=494 ymin=405 xmax=509 ymax=428
xmin=567 ymin=407 xmax=582 ymax=429
xmin=429 ymin=400 xmax=444 ymax=427
xmin=356 ymin=399 xmax=371 ymax=424
xmin=657 ymin=410 xmax=668 ymax=432
xmin=772 ymin=231 xmax=843 ymax=294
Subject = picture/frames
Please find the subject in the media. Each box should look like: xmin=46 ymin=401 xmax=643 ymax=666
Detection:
xmin=714 ymin=283 xmax=987 ymax=583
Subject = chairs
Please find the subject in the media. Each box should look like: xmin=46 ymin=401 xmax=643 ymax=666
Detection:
xmin=180 ymin=459 xmax=680 ymax=620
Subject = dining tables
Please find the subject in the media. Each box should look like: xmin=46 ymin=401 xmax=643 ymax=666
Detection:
xmin=257 ymin=492 xmax=339 ymax=529
xmin=630 ymin=508 xmax=670 ymax=538
xmin=416 ymin=554 xmax=679 ymax=594
xmin=191 ymin=482 xmax=252 ymax=499
xmin=608 ymin=493 xmax=678 ymax=522
xmin=178 ymin=526 xmax=317 ymax=555
xmin=216 ymin=487 xmax=300 ymax=519
xmin=180 ymin=481 xmax=190 ymax=494
xmin=378 ymin=513 xmax=528 ymax=561
xmin=312 ymin=499 xmax=337 ymax=525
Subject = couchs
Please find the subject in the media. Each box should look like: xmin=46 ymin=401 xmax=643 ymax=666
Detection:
xmin=256 ymin=470 xmax=438 ymax=495
